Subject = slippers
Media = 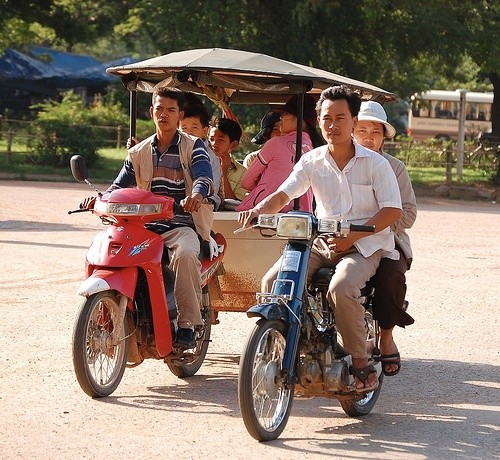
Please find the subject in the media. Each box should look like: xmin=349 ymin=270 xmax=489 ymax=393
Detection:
xmin=351 ymin=364 xmax=380 ymax=394
xmin=380 ymin=352 xmax=400 ymax=376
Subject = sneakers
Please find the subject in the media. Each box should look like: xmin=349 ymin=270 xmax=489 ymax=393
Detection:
xmin=171 ymin=328 xmax=197 ymax=348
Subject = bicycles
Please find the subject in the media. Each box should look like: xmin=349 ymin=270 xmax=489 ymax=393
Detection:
xmin=439 ymin=132 xmax=499 ymax=174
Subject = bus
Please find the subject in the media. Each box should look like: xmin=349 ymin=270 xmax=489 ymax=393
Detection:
xmin=407 ymin=90 xmax=495 ymax=134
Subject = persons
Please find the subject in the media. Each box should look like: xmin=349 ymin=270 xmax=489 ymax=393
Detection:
xmin=237 ymin=87 xmax=402 ymax=398
xmin=82 ymin=87 xmax=210 ymax=350
xmin=353 ymin=101 xmax=417 ymax=377
xmin=243 ymin=93 xmax=326 ymax=212
xmin=127 ymin=95 xmax=248 ymax=201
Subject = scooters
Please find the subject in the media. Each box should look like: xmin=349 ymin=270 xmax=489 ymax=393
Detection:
xmin=68 ymin=155 xmax=227 ymax=398
xmin=233 ymin=211 xmax=409 ymax=440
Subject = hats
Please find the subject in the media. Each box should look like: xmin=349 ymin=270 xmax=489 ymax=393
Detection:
xmin=273 ymin=93 xmax=316 ymax=129
xmin=252 ymin=111 xmax=281 ymax=144
xmin=351 ymin=100 xmax=396 ymax=138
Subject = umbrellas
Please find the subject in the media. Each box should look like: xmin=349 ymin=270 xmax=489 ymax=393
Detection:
xmin=72 ymin=55 xmax=145 ymax=85
xmin=0 ymin=45 xmax=72 ymax=83
